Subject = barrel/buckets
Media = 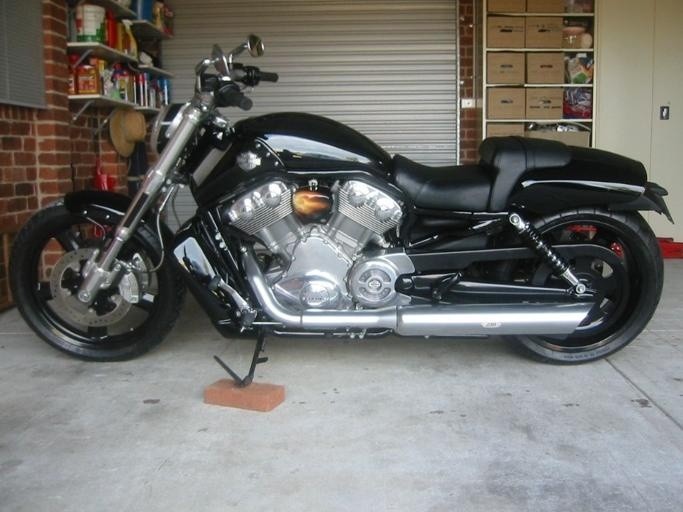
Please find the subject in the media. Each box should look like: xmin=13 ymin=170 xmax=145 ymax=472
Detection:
xmin=74 ymin=5 xmax=106 ymax=42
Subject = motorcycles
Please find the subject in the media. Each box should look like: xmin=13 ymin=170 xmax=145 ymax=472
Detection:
xmin=8 ymin=34 xmax=675 ymax=388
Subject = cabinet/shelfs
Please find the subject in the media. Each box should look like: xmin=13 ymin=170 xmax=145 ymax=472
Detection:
xmin=474 ymin=0 xmax=599 ymax=164
xmin=67 ymin=2 xmax=176 ymax=142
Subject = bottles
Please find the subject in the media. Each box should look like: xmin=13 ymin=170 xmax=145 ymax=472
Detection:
xmin=136 ymin=72 xmax=148 ymax=106
xmin=157 ymin=79 xmax=167 ymax=106
